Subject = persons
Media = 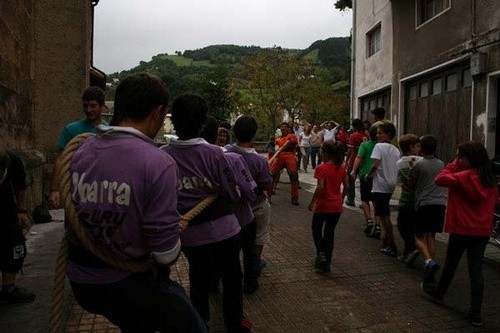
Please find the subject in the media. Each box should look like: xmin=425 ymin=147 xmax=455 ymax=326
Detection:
xmin=205 ymin=107 xmax=400 ymax=294
xmin=64 ymin=72 xmax=209 ymax=333
xmin=0 ymin=146 xmax=36 ymax=304
xmin=408 ymin=136 xmax=446 ymax=272
xmin=158 ymin=93 xmax=256 ymax=333
xmin=48 ymin=86 xmax=108 ymax=209
xmin=421 ymin=140 xmax=500 ymax=326
xmin=396 ymin=134 xmax=423 ymax=264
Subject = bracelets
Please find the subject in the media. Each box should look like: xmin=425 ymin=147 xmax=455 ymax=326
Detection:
xmin=17 ymin=208 xmax=27 ymax=213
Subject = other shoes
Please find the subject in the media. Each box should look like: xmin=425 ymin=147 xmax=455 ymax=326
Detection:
xmin=397 ymin=255 xmax=405 ymax=262
xmin=380 ymin=237 xmax=398 ymax=254
xmin=315 ymin=251 xmax=331 ymax=273
xmin=468 ymin=307 xmax=483 ymax=325
xmin=371 ymin=226 xmax=381 ymax=238
xmin=433 ymin=284 xmax=447 ymax=303
xmin=422 ymin=257 xmax=441 ymax=298
xmin=292 ymin=200 xmax=298 ymax=205
xmin=404 ymin=250 xmax=419 ymax=265
xmin=0 ymin=283 xmax=35 ymax=304
xmin=244 ymin=279 xmax=258 ymax=294
xmin=259 ymin=258 xmax=268 ymax=267
xmin=349 ymin=200 xmax=355 ymax=206
xmin=363 ymin=219 xmax=374 ymax=232
xmin=240 ymin=318 xmax=256 ymax=333
